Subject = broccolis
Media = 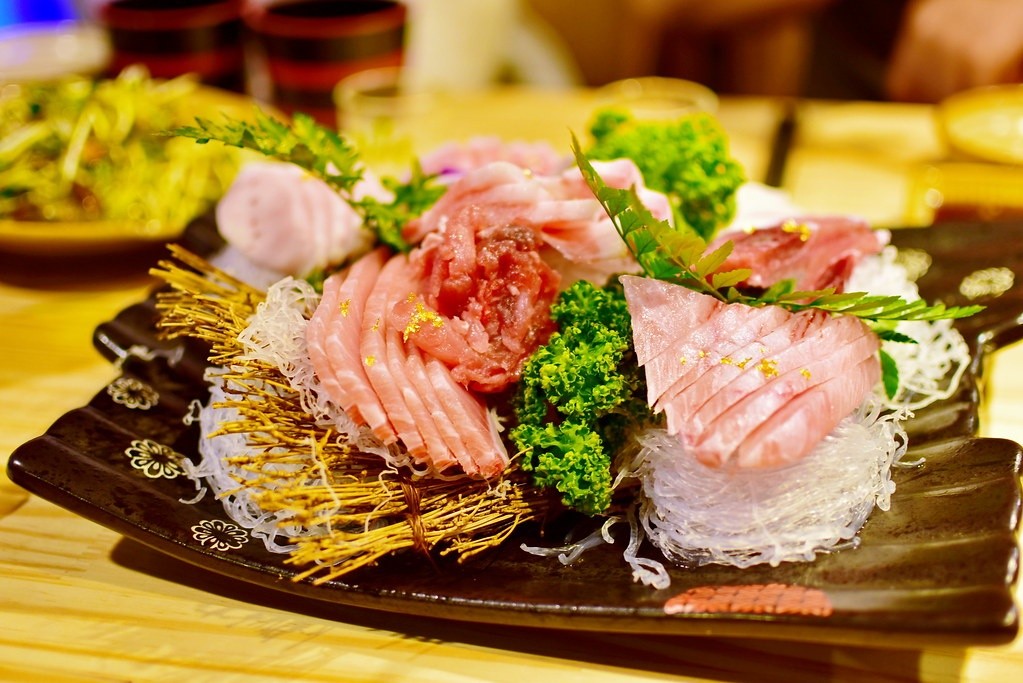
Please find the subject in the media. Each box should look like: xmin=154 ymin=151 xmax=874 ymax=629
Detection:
xmin=362 ymin=113 xmax=744 ymax=513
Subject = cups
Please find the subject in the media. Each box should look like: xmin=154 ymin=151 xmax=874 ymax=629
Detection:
xmin=245 ymin=0 xmax=406 ymax=133
xmin=100 ymin=1 xmax=250 ymax=96
xmin=335 ymin=67 xmax=436 ymax=158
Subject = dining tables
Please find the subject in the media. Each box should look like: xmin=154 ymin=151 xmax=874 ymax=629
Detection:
xmin=0 ymin=99 xmax=1022 ymax=682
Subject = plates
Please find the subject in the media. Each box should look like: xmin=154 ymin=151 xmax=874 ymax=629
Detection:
xmin=0 ymin=91 xmax=295 ymax=254
xmin=5 ymin=335 xmax=1023 ymax=641
xmin=940 ymin=83 xmax=1023 ymax=163
xmin=91 ymin=223 xmax=1023 ymax=435
